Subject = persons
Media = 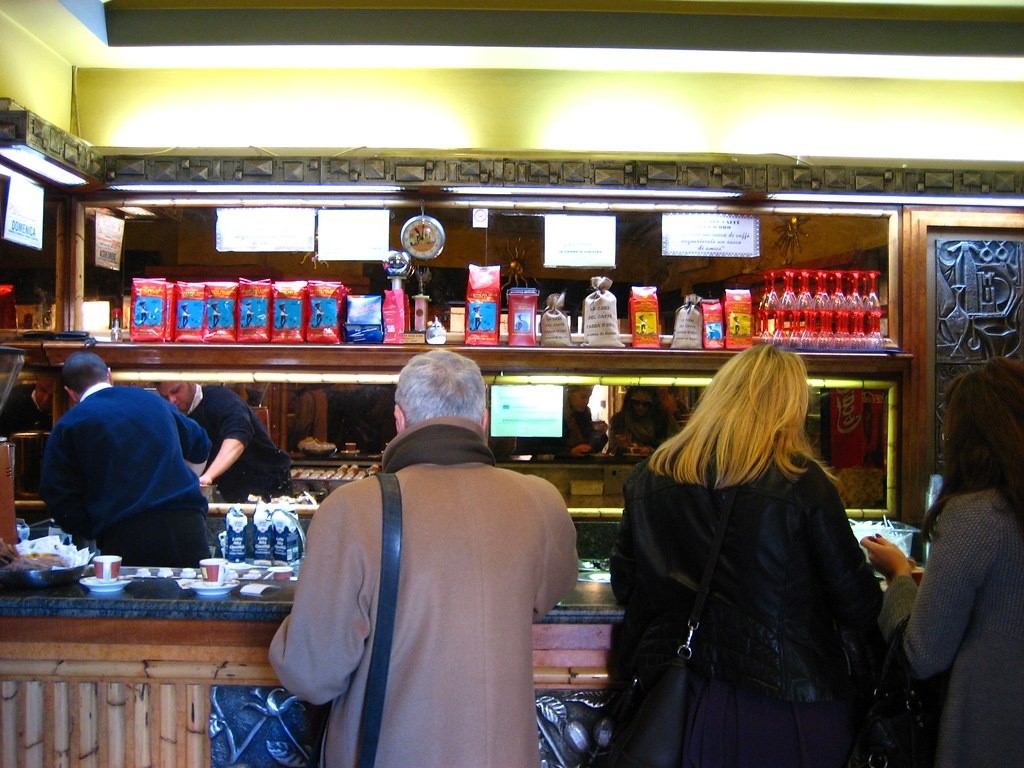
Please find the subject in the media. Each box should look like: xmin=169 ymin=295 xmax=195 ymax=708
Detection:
xmin=610 ymin=342 xmax=886 ymax=768
xmin=39 ymin=353 xmax=212 ymax=566
xmin=269 ymin=347 xmax=576 ymax=768
xmin=862 ymin=356 xmax=1024 ymax=768
xmin=608 ymin=386 xmax=681 ymax=458
xmin=0 ymin=365 xmax=58 ymax=436
xmin=549 ymin=385 xmax=608 ymax=456
xmin=296 ymin=383 xmax=357 ymax=444
xmin=152 ymin=381 xmax=293 ymax=501
xmin=669 ymin=386 xmax=690 ymax=415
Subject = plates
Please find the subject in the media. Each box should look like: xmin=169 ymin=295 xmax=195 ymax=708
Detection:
xmin=186 ymin=584 xmax=239 ymax=595
xmin=79 ymin=577 xmax=132 ymax=593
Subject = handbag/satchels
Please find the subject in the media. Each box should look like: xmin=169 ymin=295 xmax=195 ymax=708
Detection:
xmin=610 ymin=656 xmax=693 ymax=768
xmin=845 ymin=619 xmax=939 ymax=768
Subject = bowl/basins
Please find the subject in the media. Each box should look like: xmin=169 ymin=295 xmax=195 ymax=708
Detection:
xmin=340 ymin=451 xmax=360 ymax=458
xmin=0 ymin=564 xmax=87 ymax=589
xmin=301 ymin=448 xmax=337 ymax=457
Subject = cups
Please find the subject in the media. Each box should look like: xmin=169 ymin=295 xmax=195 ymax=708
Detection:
xmin=925 ymin=474 xmax=943 ymax=564
xmin=267 ymin=566 xmax=294 ymax=581
xmin=93 ymin=555 xmax=122 ymax=584
xmin=345 ymin=443 xmax=356 ymax=451
xmin=199 ymin=558 xmax=229 ymax=587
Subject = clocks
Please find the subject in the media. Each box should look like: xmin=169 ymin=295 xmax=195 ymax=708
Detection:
xmin=401 ymin=216 xmax=445 ymax=260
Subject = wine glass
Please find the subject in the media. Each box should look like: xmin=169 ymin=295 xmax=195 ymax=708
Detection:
xmin=752 ymin=268 xmax=886 ymax=350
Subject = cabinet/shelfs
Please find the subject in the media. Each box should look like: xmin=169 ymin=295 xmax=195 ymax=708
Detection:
xmin=47 ymin=189 xmax=901 ymax=548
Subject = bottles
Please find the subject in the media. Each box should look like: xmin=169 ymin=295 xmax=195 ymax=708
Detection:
xmin=110 ymin=318 xmax=122 ymax=342
